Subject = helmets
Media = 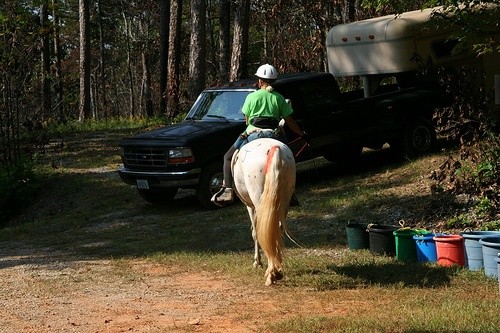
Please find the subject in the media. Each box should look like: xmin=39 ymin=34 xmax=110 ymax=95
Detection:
xmin=254 ymin=64 xmax=277 ymax=79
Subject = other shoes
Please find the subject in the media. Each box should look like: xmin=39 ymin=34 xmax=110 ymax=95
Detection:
xmin=210 ymin=188 xmax=234 ymax=202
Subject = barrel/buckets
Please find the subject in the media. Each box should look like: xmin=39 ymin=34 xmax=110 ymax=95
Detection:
xmin=413 ymin=232 xmax=447 ymax=266
xmin=432 ymin=235 xmax=465 ymax=268
xmin=478 ymin=234 xmax=500 ymax=294
xmin=393 ymin=229 xmax=428 ymax=262
xmin=366 ymin=223 xmax=404 ymax=256
xmin=345 ymin=219 xmax=369 ymax=252
xmin=457 ymin=230 xmax=500 ymax=271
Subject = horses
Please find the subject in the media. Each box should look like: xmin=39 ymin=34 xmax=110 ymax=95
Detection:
xmin=231 ymin=98 xmax=296 ymax=287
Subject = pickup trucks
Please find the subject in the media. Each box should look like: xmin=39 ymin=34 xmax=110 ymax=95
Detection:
xmin=116 ymin=71 xmax=438 ymax=209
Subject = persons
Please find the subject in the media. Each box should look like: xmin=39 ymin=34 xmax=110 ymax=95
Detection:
xmin=217 ymin=63 xmax=311 ymax=203
